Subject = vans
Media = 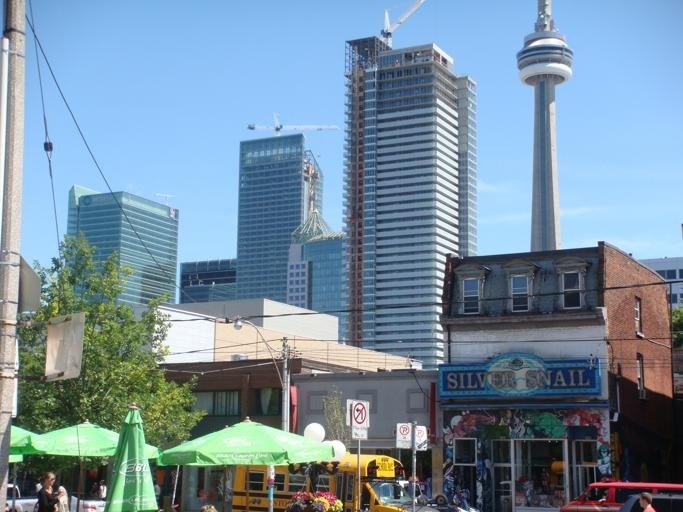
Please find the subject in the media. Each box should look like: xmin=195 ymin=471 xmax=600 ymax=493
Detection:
xmin=560 ymin=477 xmax=683 ymax=512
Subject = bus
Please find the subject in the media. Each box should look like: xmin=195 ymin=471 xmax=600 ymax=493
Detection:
xmin=231 ymin=452 xmax=440 ymax=512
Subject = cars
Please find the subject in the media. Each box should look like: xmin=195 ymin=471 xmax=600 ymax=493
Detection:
xmin=69 ymin=492 xmax=106 ymax=512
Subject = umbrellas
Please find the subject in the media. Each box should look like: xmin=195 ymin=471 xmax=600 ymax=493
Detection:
xmin=103 ymin=402 xmax=158 ymax=512
xmin=7 ymin=425 xmax=51 ymax=454
xmin=156 ymin=417 xmax=335 ymax=512
xmin=35 ymin=417 xmax=161 ymax=498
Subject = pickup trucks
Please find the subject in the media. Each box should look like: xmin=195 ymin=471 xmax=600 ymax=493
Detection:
xmin=6 ymin=484 xmax=38 ymax=512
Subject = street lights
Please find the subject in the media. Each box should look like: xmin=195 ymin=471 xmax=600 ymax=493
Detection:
xmin=234 ymin=316 xmax=291 ymax=431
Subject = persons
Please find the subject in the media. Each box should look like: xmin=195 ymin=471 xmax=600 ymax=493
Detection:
xmin=98 ymin=479 xmax=107 ymax=501
xmin=35 ymin=480 xmax=43 ymax=498
xmin=598 ymin=489 xmax=609 ymax=502
xmin=69 ymin=496 xmax=97 ymax=512
xmin=639 ymin=493 xmax=656 ymax=508
xmin=200 ymin=504 xmax=218 ymax=512
xmin=154 ymin=480 xmax=160 ymax=503
xmin=37 ymin=472 xmax=64 ymax=512
